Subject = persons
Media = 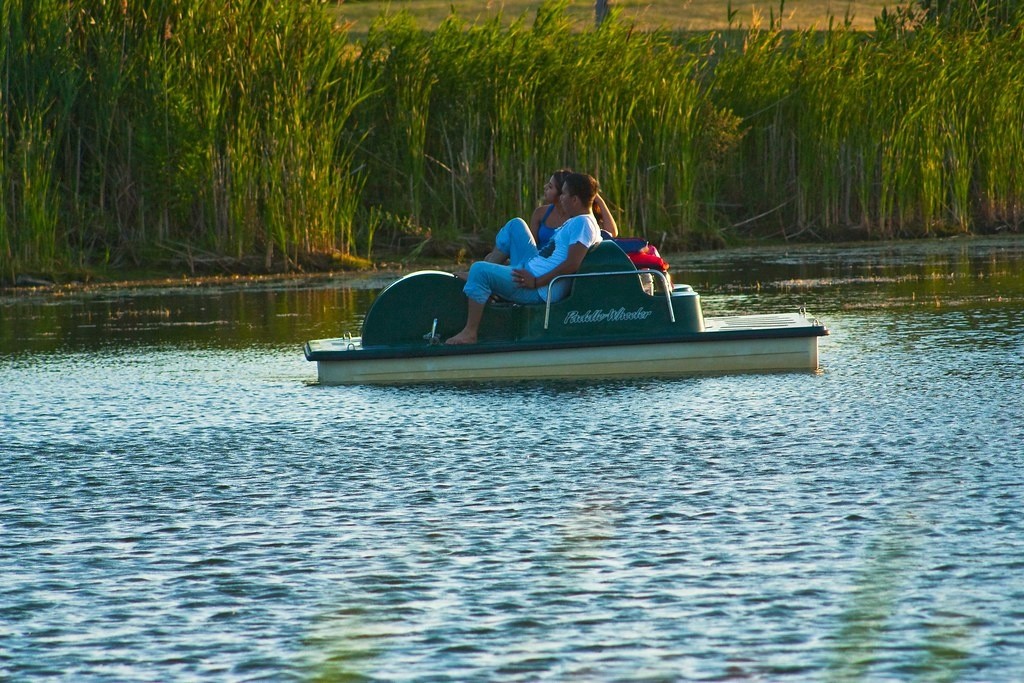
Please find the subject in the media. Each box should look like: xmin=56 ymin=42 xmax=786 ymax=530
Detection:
xmin=445 ymin=172 xmax=600 ymax=346
xmin=530 ymin=168 xmax=618 ymax=252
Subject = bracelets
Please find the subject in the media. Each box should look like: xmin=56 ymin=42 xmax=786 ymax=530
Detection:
xmin=534 ymin=276 xmax=537 ymax=289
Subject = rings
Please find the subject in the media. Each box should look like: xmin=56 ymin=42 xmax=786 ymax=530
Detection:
xmin=520 ymin=277 xmax=524 ymax=282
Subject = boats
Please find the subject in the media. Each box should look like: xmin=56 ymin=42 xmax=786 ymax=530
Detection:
xmin=300 ymin=236 xmax=832 ymax=380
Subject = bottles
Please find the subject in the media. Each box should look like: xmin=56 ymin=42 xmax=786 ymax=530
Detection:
xmin=640 ymin=267 xmax=654 ymax=297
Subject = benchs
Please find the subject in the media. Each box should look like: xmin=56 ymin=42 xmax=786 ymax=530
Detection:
xmin=515 ymin=231 xmax=642 ymax=302
